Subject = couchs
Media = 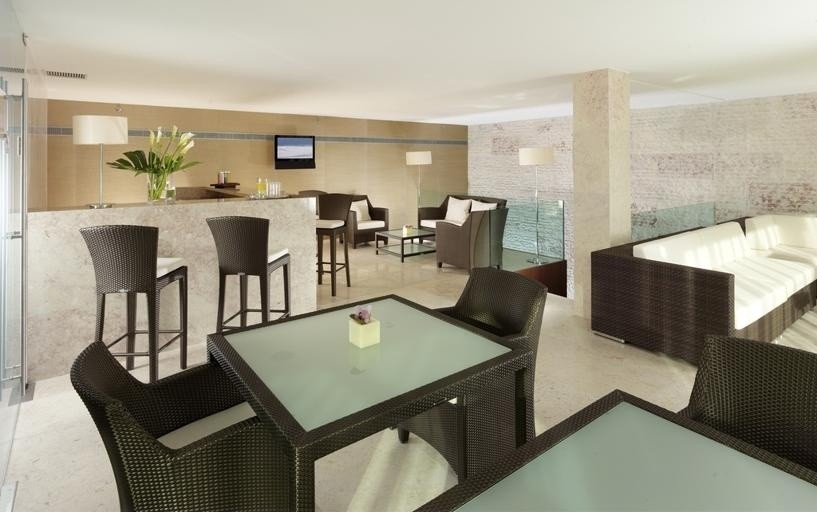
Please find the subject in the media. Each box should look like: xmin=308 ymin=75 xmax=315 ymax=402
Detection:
xmin=417 ymin=194 xmax=507 ymax=245
xmin=590 ymin=213 xmax=817 ymax=367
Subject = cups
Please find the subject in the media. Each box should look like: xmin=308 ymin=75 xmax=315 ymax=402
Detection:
xmin=273 ymin=182 xmax=280 ymax=194
xmin=165 ymin=181 xmax=175 ymax=205
xmin=270 ymin=182 xmax=273 ymax=195
xmin=256 ymin=175 xmax=268 ymax=199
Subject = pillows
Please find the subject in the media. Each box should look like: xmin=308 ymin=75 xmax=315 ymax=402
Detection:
xmin=350 ymin=199 xmax=371 ymax=221
xmin=471 ymin=200 xmax=497 ymax=211
xmin=445 ymin=196 xmax=471 ymax=224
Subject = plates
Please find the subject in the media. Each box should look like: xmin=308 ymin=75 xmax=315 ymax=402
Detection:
xmin=254 ymin=194 xmax=289 ymax=199
xmin=210 ymin=183 xmax=240 ymax=188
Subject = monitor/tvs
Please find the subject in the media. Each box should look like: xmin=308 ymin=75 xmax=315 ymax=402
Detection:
xmin=274 ymin=135 xmax=316 ymax=170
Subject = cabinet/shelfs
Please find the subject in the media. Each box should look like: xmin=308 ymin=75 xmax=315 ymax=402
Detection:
xmin=23 ymin=185 xmax=316 ymax=384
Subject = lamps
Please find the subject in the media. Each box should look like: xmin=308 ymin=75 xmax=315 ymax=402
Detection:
xmin=405 ymin=151 xmax=432 ymax=166
xmin=72 ymin=114 xmax=128 ymax=209
xmin=519 ymin=147 xmax=555 ymax=166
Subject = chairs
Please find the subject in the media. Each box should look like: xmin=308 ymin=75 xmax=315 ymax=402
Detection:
xmin=436 ymin=208 xmax=509 ymax=271
xmin=205 ymin=215 xmax=291 ymax=332
xmin=339 ymin=195 xmax=389 ymax=249
xmin=676 ymin=333 xmax=817 ymax=468
xmin=70 ymin=339 xmax=294 ymax=512
xmin=398 ymin=265 xmax=549 ymax=475
xmin=314 ymin=193 xmax=353 ymax=296
xmin=79 ymin=226 xmax=188 ymax=381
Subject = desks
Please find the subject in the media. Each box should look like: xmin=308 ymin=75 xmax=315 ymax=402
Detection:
xmin=415 ymin=389 xmax=817 ymax=512
xmin=207 ymin=292 xmax=535 ymax=512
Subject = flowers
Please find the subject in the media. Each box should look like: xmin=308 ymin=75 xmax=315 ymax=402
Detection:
xmin=106 ymin=124 xmax=202 ymax=201
